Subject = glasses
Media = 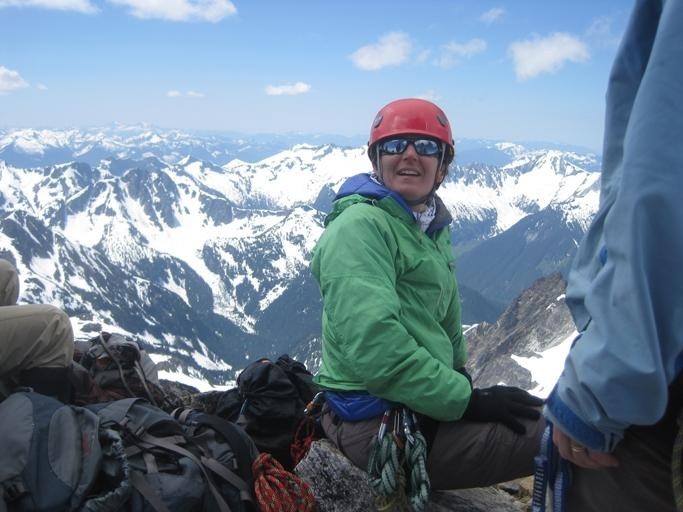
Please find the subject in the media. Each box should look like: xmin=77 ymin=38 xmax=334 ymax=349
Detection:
xmin=379 ymin=137 xmax=442 ymax=155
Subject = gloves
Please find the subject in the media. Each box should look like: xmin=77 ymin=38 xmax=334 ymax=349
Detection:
xmin=462 ymin=385 xmax=543 ymax=435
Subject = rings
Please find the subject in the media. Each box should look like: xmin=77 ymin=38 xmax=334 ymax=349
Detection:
xmin=571 ymin=446 xmax=588 ymax=457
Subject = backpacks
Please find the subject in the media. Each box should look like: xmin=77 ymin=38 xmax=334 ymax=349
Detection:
xmin=1 ymin=303 xmax=321 ymax=511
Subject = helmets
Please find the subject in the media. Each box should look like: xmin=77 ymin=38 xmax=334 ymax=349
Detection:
xmin=367 ymin=98 xmax=454 ymax=165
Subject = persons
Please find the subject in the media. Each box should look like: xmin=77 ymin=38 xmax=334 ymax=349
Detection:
xmin=311 ymin=97 xmax=547 ymax=490
xmin=0 ymin=258 xmax=73 ymax=404
xmin=541 ymin=0 xmax=682 ymax=508
